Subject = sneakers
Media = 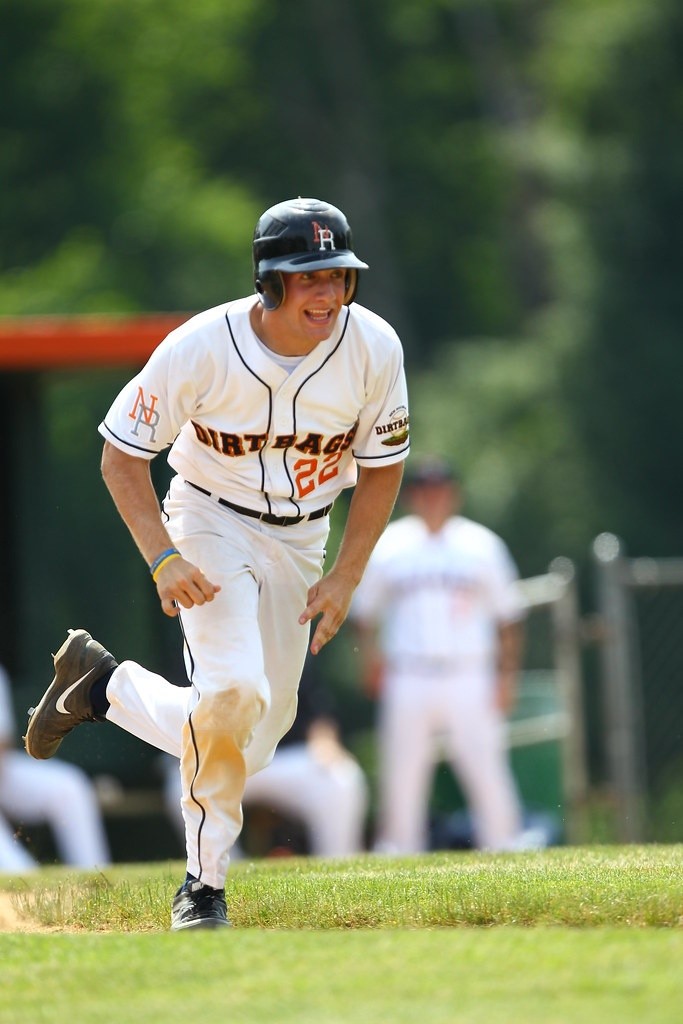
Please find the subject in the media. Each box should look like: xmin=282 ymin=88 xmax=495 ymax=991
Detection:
xmin=22 ymin=628 xmax=119 ymax=761
xmin=171 ymin=879 xmax=231 ymax=933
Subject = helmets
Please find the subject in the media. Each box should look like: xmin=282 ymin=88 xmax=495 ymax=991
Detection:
xmin=253 ymin=198 xmax=369 ymax=312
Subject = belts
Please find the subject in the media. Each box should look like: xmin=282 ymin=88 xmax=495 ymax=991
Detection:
xmin=187 ymin=481 xmax=332 ymax=527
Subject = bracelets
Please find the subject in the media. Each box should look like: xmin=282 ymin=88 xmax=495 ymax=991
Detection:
xmin=150 ymin=548 xmax=181 ymax=581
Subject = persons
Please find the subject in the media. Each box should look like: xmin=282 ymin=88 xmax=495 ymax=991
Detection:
xmin=22 ymin=198 xmax=411 ymax=933
xmin=346 ymin=448 xmax=548 ymax=857
xmin=162 ymin=634 xmax=373 ymax=861
xmin=1 ymin=666 xmax=112 ymax=874
